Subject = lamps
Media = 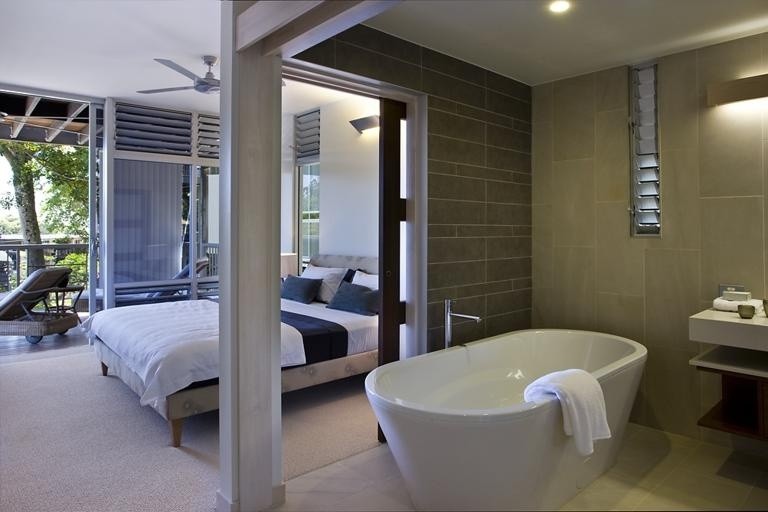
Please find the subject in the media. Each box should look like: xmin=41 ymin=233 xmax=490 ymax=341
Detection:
xmin=347 ymin=110 xmax=380 ymax=135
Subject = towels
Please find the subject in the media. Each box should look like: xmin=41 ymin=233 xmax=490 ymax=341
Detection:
xmin=525 ymin=366 xmax=613 ymax=458
xmin=712 ymin=296 xmax=764 ymax=314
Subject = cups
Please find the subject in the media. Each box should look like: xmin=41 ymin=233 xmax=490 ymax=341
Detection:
xmin=738 ymin=305 xmax=755 ymax=319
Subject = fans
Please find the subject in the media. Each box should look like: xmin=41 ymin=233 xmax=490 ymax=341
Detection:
xmin=136 ymin=56 xmax=287 ymax=99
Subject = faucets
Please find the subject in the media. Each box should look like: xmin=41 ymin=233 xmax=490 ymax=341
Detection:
xmin=442 ymin=295 xmax=484 ymax=350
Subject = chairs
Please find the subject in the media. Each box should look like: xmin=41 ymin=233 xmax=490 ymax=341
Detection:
xmin=0 ymin=258 xmax=210 ymax=344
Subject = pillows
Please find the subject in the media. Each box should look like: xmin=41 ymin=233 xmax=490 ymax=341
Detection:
xmin=282 ymin=263 xmax=380 ymax=317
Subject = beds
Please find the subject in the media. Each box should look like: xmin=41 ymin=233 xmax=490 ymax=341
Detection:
xmin=83 ymin=254 xmax=414 ymax=447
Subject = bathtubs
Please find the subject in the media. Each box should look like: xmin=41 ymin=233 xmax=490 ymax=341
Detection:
xmin=365 ymin=325 xmax=649 ymax=512
